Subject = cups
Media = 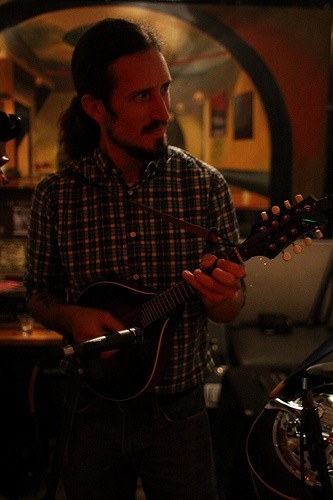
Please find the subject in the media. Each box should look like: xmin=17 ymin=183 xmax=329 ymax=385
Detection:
xmin=17 ymin=314 xmax=34 ymax=332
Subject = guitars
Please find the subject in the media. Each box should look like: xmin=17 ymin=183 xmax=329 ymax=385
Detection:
xmin=62 ymin=194 xmax=327 ymax=403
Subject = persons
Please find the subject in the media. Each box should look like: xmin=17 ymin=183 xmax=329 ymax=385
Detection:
xmin=24 ymin=18 xmax=247 ymax=500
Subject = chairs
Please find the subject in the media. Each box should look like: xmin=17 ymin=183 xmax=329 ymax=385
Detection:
xmin=228 ymin=260 xmax=333 ymax=371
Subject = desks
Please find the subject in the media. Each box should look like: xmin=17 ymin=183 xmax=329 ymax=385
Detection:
xmin=0 ymin=317 xmax=63 ymax=348
